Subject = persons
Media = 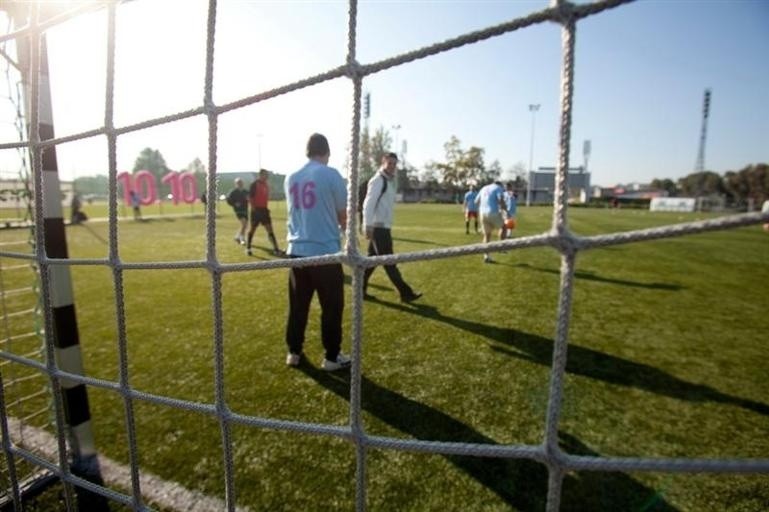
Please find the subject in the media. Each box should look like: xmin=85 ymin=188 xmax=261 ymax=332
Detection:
xmin=462 ymin=180 xmax=517 ymax=262
xmin=245 ymin=169 xmax=280 ymax=256
xmin=285 ymin=132 xmax=351 ymax=372
xmin=362 ymin=152 xmax=422 ymax=303
xmin=71 ymin=193 xmax=80 ymax=223
xmin=129 ymin=190 xmax=141 ymax=217
xmin=226 ymin=178 xmax=250 ymax=244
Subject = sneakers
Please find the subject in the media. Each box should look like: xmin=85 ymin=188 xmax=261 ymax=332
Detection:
xmin=286 ymin=348 xmax=305 ymax=366
xmin=401 ymin=290 xmax=423 ymax=305
xmin=322 ymin=353 xmax=352 ymax=374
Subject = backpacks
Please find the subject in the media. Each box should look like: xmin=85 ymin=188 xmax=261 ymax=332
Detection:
xmin=358 ymin=173 xmax=388 ymax=222
